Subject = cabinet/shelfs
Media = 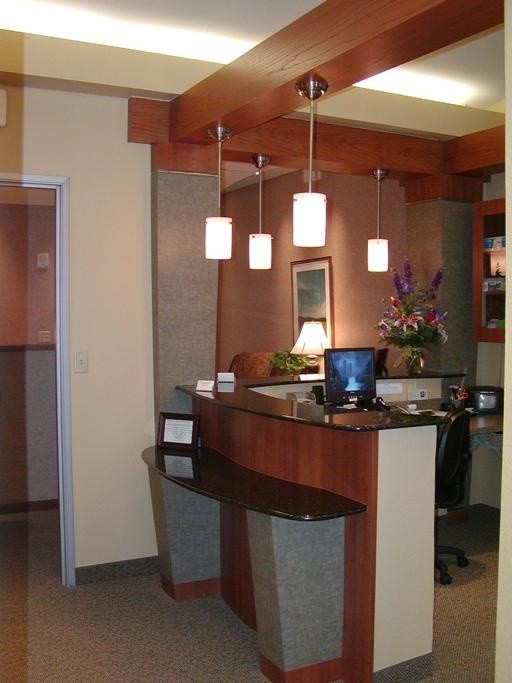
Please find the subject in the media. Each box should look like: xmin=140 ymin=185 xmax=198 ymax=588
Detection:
xmin=472 ymin=196 xmax=506 ymax=344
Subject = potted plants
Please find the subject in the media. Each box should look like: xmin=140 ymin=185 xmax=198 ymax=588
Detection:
xmin=269 ymin=343 xmax=308 ymax=376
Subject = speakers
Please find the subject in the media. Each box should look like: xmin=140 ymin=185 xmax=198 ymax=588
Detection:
xmin=312 ymin=386 xmax=324 ymax=405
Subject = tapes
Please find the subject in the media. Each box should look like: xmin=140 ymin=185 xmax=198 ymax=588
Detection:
xmin=380 ymin=400 xmax=385 ymax=406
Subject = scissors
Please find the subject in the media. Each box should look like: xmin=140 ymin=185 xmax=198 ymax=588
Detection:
xmin=459 ymin=388 xmax=469 ymax=400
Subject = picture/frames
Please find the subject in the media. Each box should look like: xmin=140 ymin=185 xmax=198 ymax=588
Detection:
xmin=156 ymin=411 xmax=203 ymax=450
xmin=290 ymin=255 xmax=333 ymax=357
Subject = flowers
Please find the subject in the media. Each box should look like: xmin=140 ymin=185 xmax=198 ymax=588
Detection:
xmin=375 ymin=255 xmax=448 ymax=344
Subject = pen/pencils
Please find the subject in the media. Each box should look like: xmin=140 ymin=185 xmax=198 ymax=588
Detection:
xmin=449 ymin=383 xmax=464 ymax=400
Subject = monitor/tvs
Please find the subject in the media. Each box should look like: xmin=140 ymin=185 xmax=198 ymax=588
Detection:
xmin=325 ymin=346 xmax=377 ymax=411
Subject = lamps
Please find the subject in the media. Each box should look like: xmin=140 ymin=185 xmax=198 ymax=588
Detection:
xmin=201 ymin=125 xmax=235 ymax=260
xmin=401 ymin=344 xmax=425 ymax=374
xmin=365 ymin=167 xmax=392 ymax=273
xmin=247 ymin=154 xmax=273 ymax=270
xmin=290 ymin=319 xmax=332 ymax=373
xmin=289 ymin=70 xmax=333 ymax=247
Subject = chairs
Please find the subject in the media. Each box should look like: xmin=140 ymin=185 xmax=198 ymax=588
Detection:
xmin=434 ymin=408 xmax=476 ymax=586
